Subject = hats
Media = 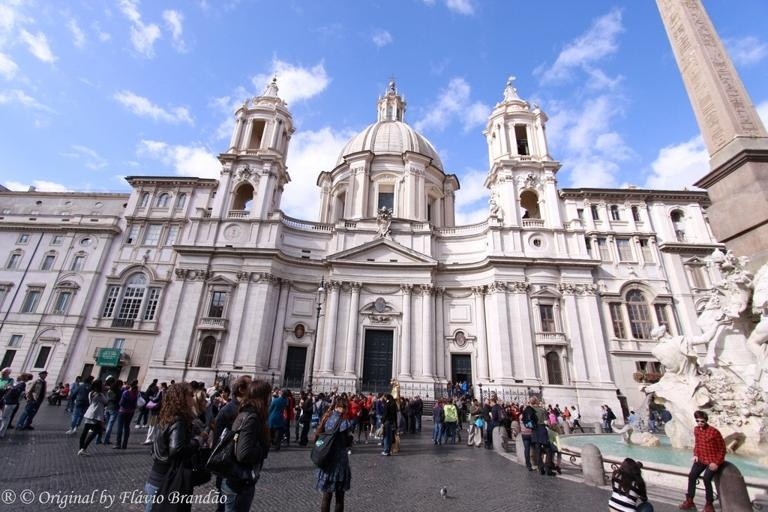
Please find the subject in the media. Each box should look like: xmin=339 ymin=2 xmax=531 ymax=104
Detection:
xmin=38 ymin=371 xmax=48 ymax=374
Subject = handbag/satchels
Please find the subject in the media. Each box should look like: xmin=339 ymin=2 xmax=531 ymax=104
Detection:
xmin=497 ymin=408 xmax=510 ymax=423
xmin=207 ymin=412 xmax=265 ymax=480
xmin=145 ymin=399 xmax=159 ymax=410
xmin=375 ymin=424 xmax=385 ymax=437
xmin=311 ymin=430 xmax=341 ymax=472
xmin=475 ymin=415 xmax=485 ymax=428
xmin=135 ymin=392 xmax=146 ymax=410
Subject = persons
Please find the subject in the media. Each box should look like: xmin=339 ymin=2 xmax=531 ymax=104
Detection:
xmin=608 ymin=458 xmax=654 ymax=512
xmin=377 ymin=206 xmax=391 ymax=237
xmin=679 ymin=410 xmax=726 ymax=512
xmin=600 ymin=402 xmax=672 ymax=434
xmin=650 ymin=321 xmax=732 ymax=406
xmin=522 ymin=210 xmax=531 ymax=219
xmin=745 ymin=263 xmax=768 ymax=372
xmin=0 ymin=365 xmax=585 ymax=511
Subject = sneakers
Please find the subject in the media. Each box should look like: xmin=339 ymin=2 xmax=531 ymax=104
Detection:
xmin=527 ymin=463 xmax=562 ymax=477
xmin=679 ymin=498 xmax=694 ymax=509
xmin=7 ymin=422 xmax=154 ymax=458
xmin=704 ymin=504 xmax=714 ymax=512
xmin=347 ymin=439 xmax=390 ymax=457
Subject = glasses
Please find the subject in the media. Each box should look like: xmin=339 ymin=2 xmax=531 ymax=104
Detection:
xmin=695 ymin=420 xmax=705 ymax=423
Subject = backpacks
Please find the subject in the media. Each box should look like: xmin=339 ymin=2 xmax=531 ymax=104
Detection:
xmin=522 ymin=405 xmax=539 ymax=430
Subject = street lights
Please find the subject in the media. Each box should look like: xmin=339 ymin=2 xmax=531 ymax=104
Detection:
xmin=307 ymin=285 xmax=328 ymax=395
